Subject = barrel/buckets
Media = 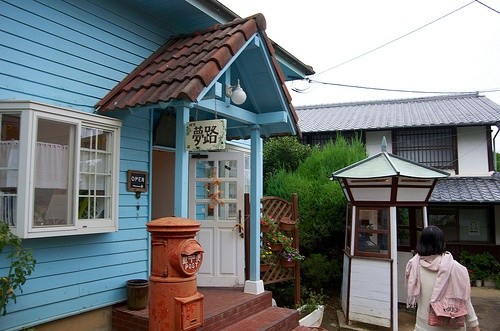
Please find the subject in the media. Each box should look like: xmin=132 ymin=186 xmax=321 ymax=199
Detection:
xmin=125 ymin=279 xmax=149 ymax=310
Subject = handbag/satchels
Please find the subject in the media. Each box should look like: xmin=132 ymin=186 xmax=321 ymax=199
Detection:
xmin=428 ymin=302 xmax=469 ymax=328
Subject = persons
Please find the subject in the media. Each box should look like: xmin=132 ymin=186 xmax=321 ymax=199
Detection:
xmin=405 ymin=226 xmax=481 ymax=331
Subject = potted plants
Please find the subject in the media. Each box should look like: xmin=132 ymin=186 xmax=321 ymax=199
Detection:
xmin=284 ymin=287 xmax=329 ymax=327
xmin=475 ymin=270 xmax=487 ymax=287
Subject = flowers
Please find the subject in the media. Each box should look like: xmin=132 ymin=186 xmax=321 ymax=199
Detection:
xmin=260 ymin=213 xmax=278 ymax=233
xmin=264 ymin=231 xmax=292 ymax=249
xmin=280 ymin=246 xmax=305 ymax=262
xmin=260 ymin=243 xmax=277 ymax=269
xmin=468 ymin=269 xmax=476 ymax=280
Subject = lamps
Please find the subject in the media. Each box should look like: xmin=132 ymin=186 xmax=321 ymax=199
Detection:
xmin=225 ymin=79 xmax=247 ymax=105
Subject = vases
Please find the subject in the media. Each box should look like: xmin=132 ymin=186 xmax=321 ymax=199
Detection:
xmin=281 ymin=259 xmax=295 ymax=267
xmin=261 ymin=224 xmax=271 ymax=232
xmin=470 ymin=280 xmax=472 ymax=287
xmin=260 ymin=264 xmax=269 ymax=272
xmin=281 ymin=221 xmax=295 ymax=231
xmin=271 ymin=243 xmax=282 ymax=251
